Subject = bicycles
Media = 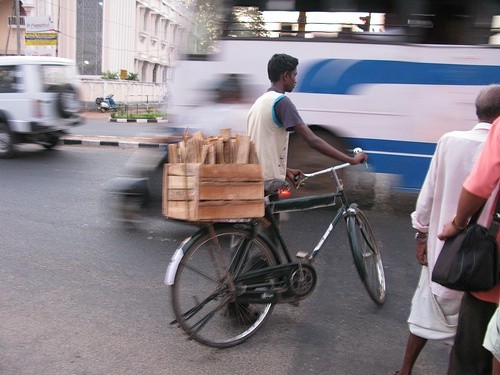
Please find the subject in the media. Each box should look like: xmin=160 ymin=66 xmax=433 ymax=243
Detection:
xmin=164 ymin=147 xmax=387 ymax=349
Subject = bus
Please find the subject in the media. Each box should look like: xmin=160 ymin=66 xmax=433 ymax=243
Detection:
xmin=167 ymin=0 xmax=500 ymax=203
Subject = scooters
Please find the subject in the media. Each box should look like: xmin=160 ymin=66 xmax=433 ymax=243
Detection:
xmin=97 ymin=94 xmax=119 ymax=112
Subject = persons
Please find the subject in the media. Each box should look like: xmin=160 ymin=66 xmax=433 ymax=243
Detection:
xmin=438 ymin=116 xmax=500 ymax=375
xmin=224 ymin=54 xmax=370 ymax=324
xmin=396 ymin=83 xmax=500 ymax=375
xmin=482 ymin=304 xmax=500 ymax=375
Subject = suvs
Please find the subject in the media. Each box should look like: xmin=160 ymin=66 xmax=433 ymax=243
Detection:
xmin=0 ymin=52 xmax=82 ymax=158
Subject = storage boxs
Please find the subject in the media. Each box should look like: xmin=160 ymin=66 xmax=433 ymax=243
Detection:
xmin=160 ymin=161 xmax=265 ymax=223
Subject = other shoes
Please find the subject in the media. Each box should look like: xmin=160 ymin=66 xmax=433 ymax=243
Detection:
xmin=226 ymin=310 xmax=260 ymax=324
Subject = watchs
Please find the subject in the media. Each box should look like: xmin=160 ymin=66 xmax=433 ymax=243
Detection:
xmin=414 ymin=232 xmax=426 ymax=243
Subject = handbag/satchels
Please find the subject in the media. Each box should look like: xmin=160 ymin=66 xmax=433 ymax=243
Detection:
xmin=430 ymin=223 xmax=498 ymax=292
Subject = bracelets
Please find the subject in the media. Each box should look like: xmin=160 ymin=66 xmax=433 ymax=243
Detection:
xmin=452 ymin=218 xmax=464 ymax=231
